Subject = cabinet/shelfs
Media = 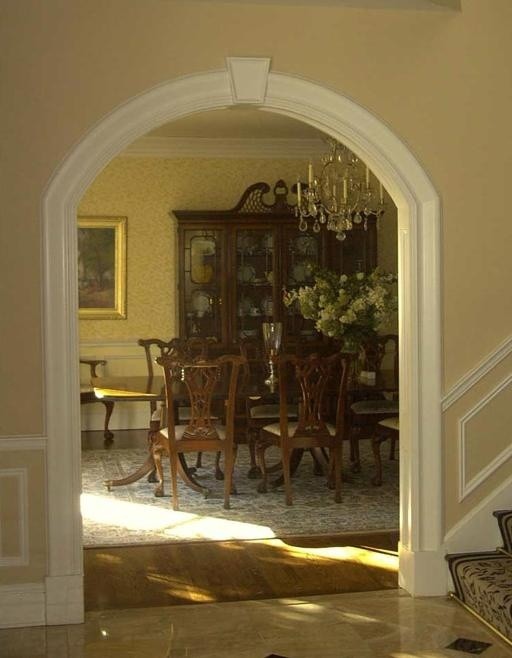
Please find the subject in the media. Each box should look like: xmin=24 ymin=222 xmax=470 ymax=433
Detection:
xmin=169 ymin=177 xmax=386 ymax=418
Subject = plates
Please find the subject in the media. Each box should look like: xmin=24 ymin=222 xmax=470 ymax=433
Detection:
xmin=185 ymin=229 xmax=321 ymax=345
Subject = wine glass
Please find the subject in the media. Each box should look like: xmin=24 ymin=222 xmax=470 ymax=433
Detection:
xmin=261 ymin=319 xmax=284 ymax=385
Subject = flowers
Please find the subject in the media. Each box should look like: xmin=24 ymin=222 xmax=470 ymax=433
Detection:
xmin=277 ymin=260 xmax=399 ymax=344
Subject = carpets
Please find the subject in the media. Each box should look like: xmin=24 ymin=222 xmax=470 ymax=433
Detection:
xmin=80 ymin=436 xmax=400 ymax=550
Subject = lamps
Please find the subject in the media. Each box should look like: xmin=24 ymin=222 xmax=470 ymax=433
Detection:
xmin=292 ymin=132 xmax=387 ymax=245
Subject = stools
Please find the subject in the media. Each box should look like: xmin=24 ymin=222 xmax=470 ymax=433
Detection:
xmin=369 ymin=414 xmax=403 ymax=486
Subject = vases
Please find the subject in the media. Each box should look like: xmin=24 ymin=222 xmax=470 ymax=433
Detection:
xmin=337 ymin=334 xmax=367 ymax=377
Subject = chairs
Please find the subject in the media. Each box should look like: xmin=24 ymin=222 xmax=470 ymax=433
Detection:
xmin=133 ymin=332 xmax=225 ymax=481
xmin=257 ymin=348 xmax=357 ymax=508
xmin=81 ymin=355 xmax=119 ymax=443
xmin=151 ymin=349 xmax=248 ymax=513
xmin=237 ymin=332 xmax=310 ymax=477
xmin=346 ymin=331 xmax=403 ymax=474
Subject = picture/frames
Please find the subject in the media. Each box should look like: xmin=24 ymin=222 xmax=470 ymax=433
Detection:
xmin=77 ymin=213 xmax=131 ymax=322
